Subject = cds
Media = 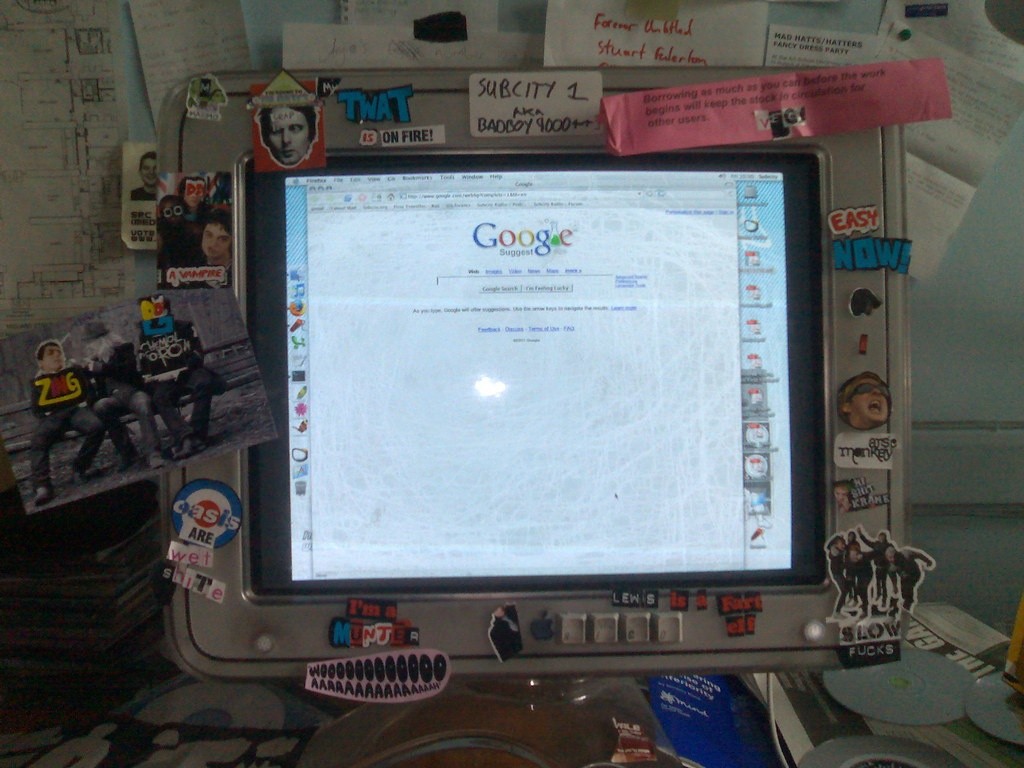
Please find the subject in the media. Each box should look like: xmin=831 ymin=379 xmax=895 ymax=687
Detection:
xmin=965 ymin=671 xmax=1024 ymax=746
xmin=822 ymin=646 xmax=975 ymax=725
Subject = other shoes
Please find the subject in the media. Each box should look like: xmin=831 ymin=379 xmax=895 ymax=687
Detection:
xmin=33 ymin=487 xmax=52 ymax=504
xmin=150 ymin=454 xmax=162 ymax=468
xmin=176 ymin=439 xmax=206 ymax=457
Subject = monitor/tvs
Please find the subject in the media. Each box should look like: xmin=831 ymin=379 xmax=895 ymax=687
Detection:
xmin=157 ymin=66 xmax=907 ymax=768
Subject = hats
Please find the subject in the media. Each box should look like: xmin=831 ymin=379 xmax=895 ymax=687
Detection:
xmin=80 ymin=319 xmax=109 ymax=339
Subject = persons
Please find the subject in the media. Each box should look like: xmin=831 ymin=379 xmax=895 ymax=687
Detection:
xmin=31 ymin=99 xmax=319 ymax=506
xmin=840 ymin=371 xmax=893 ymax=432
xmin=825 ymin=526 xmax=937 ymax=622
